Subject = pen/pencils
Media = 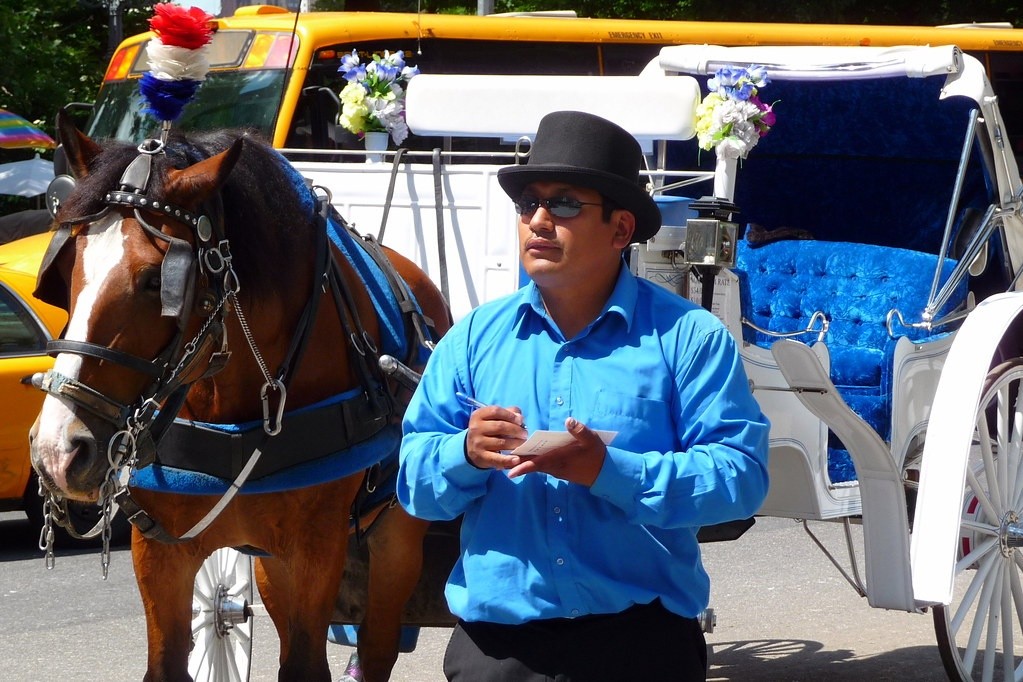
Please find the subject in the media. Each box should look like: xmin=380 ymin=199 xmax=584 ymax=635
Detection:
xmin=455 ymin=392 xmax=528 ymax=429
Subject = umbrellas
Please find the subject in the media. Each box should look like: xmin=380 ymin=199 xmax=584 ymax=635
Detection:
xmin=0 ymin=108 xmax=55 ymax=149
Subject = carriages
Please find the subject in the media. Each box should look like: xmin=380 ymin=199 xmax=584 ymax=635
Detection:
xmin=23 ymin=38 xmax=1023 ymax=682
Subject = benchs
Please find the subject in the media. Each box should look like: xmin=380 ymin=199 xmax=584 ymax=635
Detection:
xmin=737 ymin=241 xmax=972 ymax=482
xmin=392 ymin=73 xmax=711 ymax=225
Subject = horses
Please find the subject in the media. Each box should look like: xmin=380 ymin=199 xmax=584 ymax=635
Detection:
xmin=27 ymin=104 xmax=454 ymax=682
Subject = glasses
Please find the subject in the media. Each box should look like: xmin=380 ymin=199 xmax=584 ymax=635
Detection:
xmin=512 ymin=191 xmax=608 ymax=220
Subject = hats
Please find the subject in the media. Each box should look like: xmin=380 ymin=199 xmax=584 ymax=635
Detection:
xmin=496 ymin=112 xmax=662 ymax=241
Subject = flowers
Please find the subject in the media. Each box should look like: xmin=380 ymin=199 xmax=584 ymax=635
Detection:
xmin=335 ymin=45 xmax=421 ymax=147
xmin=695 ymin=59 xmax=782 ymax=153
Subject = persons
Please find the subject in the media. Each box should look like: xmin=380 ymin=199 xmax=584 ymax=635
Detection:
xmin=395 ymin=111 xmax=770 ymax=682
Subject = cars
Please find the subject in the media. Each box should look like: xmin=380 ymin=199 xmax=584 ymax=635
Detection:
xmin=0 ymin=203 xmax=131 ymax=547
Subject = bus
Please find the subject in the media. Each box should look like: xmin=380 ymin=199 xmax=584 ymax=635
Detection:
xmin=48 ymin=15 xmax=1023 ymax=198
